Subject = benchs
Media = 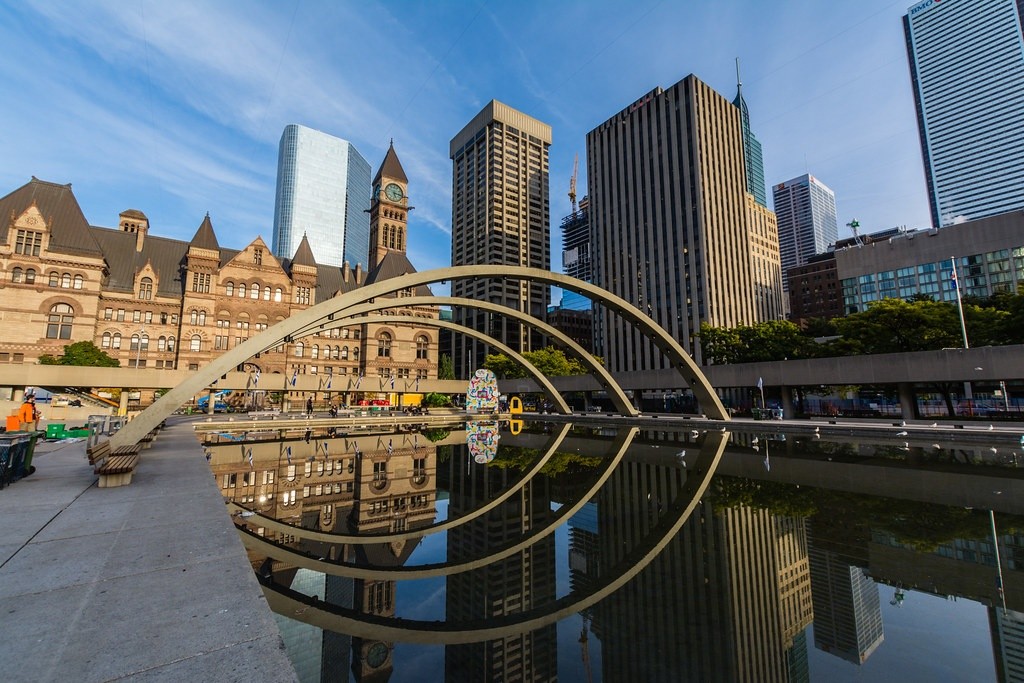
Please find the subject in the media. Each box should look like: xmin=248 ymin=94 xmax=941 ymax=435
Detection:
xmin=762 ymin=409 xmax=783 ymax=420
xmin=331 ymin=410 xmax=356 ymax=418
xmin=287 ymin=413 xmax=317 ymax=420
xmin=402 ymin=406 xmax=428 ymax=415
xmin=87 ymin=440 xmax=139 ymax=487
xmin=372 ymin=410 xmax=395 ymax=417
xmin=139 ymin=434 xmax=154 ymax=450
xmin=150 ymin=425 xmax=161 ymax=435
xmin=110 ymin=445 xmax=143 ymax=455
xmin=248 ymin=411 xmax=280 ymax=420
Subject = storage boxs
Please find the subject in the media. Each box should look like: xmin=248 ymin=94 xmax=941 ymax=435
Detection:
xmin=47 ymin=423 xmax=94 ymax=439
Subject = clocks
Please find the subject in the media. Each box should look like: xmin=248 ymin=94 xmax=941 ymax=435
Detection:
xmin=385 ymin=184 xmax=403 ymax=201
xmin=375 ymin=185 xmax=380 ymax=203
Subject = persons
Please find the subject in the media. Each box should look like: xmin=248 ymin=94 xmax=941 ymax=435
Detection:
xmin=18 ymin=394 xmax=36 ymax=431
xmin=329 ymin=405 xmax=338 ymax=418
xmin=408 ymin=403 xmax=423 ymax=416
xmin=269 ymin=404 xmax=274 ymax=411
xmin=227 ymin=405 xmax=231 ymax=413
xmin=307 ymin=397 xmax=313 ymax=414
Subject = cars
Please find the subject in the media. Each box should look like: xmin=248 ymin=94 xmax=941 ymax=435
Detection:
xmin=957 ymin=401 xmax=1003 ymax=418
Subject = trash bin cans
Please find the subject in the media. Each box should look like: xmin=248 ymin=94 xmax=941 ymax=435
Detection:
xmin=752 ymin=408 xmax=784 ymax=420
xmin=0 ymin=431 xmax=39 ymax=489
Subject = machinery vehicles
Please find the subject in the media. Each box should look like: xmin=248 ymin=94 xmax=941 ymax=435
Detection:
xmin=197 ymin=389 xmax=234 ymax=414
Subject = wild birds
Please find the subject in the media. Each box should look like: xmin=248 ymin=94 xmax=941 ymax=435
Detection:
xmin=814 ymin=421 xmax=940 ymax=450
xmin=989 ymin=424 xmax=997 ymax=454
xmin=675 ymin=426 xmax=758 ymax=457
